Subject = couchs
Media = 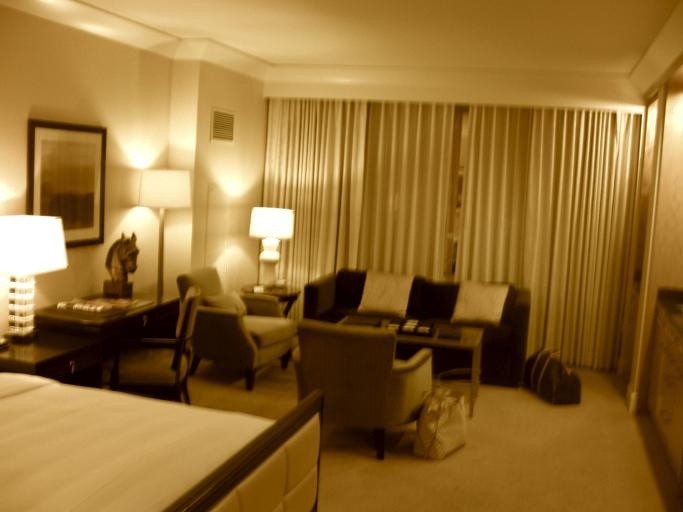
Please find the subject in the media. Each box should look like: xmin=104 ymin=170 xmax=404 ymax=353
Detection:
xmin=304 ymin=269 xmax=531 ymax=388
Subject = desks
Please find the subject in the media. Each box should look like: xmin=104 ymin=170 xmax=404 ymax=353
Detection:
xmin=336 ymin=314 xmax=484 ymax=417
xmin=35 ymin=288 xmax=182 ymax=328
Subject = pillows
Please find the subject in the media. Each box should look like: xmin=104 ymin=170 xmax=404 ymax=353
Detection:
xmin=357 ymin=269 xmax=414 ymax=321
xmin=204 ymin=292 xmax=247 ymax=315
xmin=452 ymin=281 xmax=510 ymax=327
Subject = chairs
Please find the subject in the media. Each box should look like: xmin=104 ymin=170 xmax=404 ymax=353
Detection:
xmin=178 ymin=266 xmax=297 ymax=390
xmin=114 ymin=285 xmax=202 ymax=403
xmin=297 ymin=319 xmax=434 ymax=460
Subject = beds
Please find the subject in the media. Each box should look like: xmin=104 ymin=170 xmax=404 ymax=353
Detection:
xmin=1 ymin=372 xmax=324 ymax=512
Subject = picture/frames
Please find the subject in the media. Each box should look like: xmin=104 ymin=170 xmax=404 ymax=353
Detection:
xmin=27 ymin=118 xmax=108 ymax=247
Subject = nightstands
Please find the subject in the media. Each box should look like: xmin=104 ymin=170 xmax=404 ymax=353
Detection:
xmin=1 ymin=331 xmax=102 ymax=388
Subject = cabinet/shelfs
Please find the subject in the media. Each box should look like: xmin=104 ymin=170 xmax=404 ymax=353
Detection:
xmin=645 ymin=287 xmax=683 ymax=511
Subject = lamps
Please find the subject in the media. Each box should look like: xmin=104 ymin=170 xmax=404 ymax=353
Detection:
xmin=249 ymin=206 xmax=295 ymax=288
xmin=1 ymin=215 xmax=69 ymax=344
xmin=135 ymin=168 xmax=192 ymax=302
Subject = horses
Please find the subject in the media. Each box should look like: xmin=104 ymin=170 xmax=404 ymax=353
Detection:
xmin=105 ymin=231 xmax=140 ymax=297
